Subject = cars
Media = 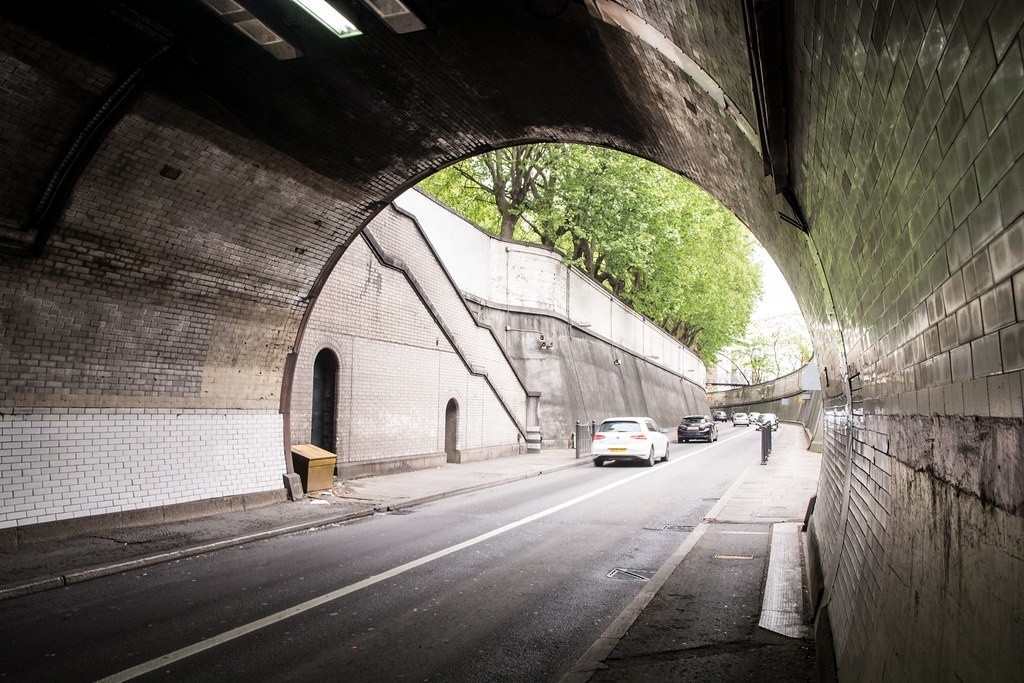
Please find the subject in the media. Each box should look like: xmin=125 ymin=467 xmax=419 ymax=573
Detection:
xmin=590 ymin=417 xmax=671 ymax=467
xmin=733 ymin=413 xmax=750 ymax=427
xmin=747 ymin=412 xmax=761 ymax=423
xmin=756 ymin=413 xmax=779 ymax=432
xmin=714 ymin=411 xmax=728 ymax=422
xmin=677 ymin=415 xmax=719 ymax=444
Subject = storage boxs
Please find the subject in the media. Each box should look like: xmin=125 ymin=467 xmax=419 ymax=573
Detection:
xmin=291 ymin=444 xmax=338 ymax=493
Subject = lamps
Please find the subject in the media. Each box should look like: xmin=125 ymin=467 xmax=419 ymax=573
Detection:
xmin=539 ymin=334 xmax=554 ymax=350
xmin=201 ymin=0 xmax=306 ymax=61
xmin=359 ymin=0 xmax=428 ymax=34
xmin=614 ymin=359 xmax=622 ymax=365
xmin=289 ymin=0 xmax=363 ymax=39
